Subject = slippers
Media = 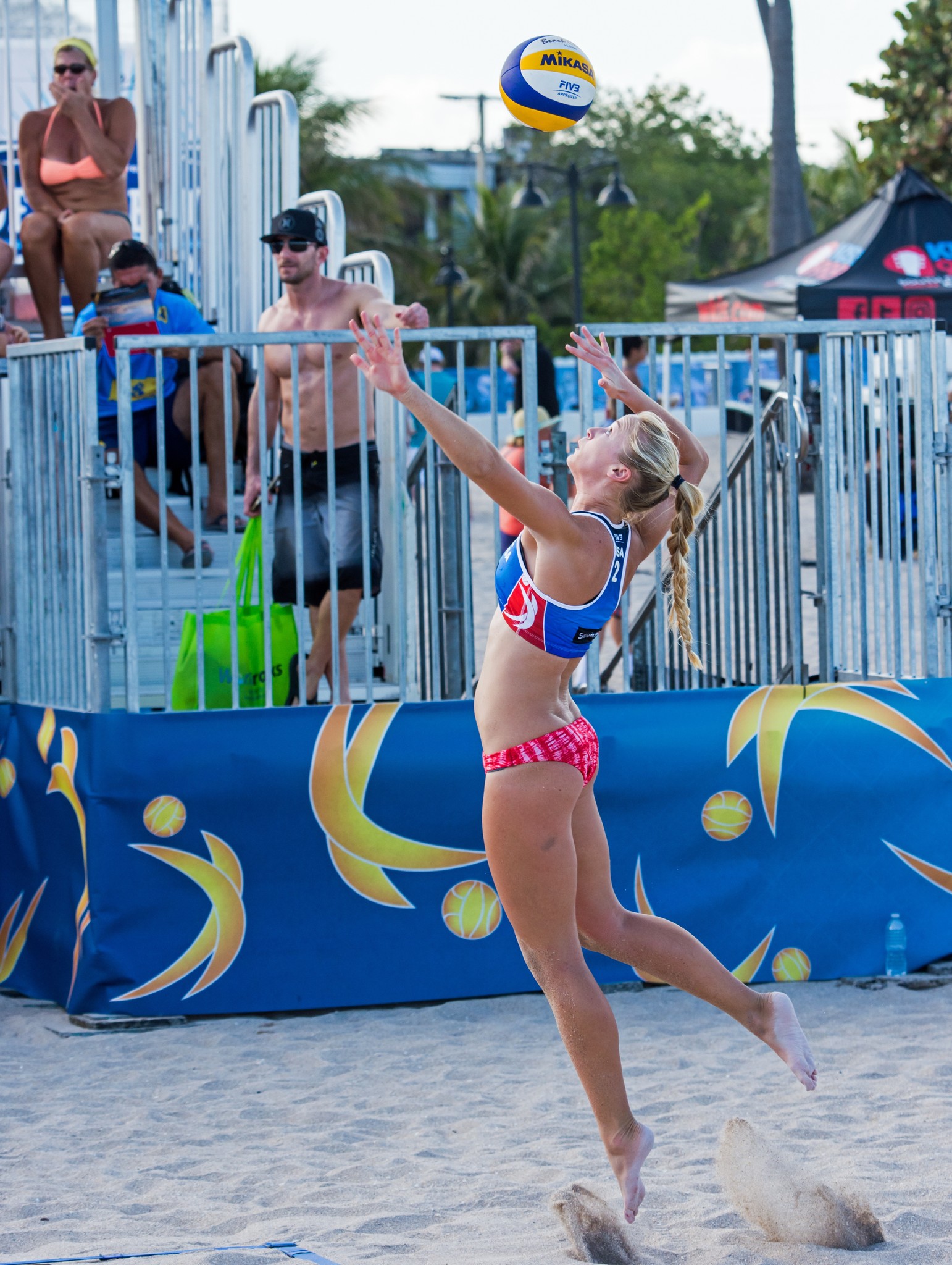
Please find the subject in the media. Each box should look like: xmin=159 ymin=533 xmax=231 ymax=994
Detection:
xmin=286 ymin=654 xmax=318 ymax=707
xmin=181 ymin=540 xmax=213 ymax=567
xmin=205 ymin=514 xmax=245 ymax=530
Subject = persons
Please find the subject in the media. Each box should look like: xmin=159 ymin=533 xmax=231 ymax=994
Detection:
xmin=864 ymin=375 xmax=916 ymax=540
xmin=0 ymin=35 xmax=257 ymax=568
xmin=243 ymin=212 xmax=430 ymax=707
xmin=349 ymin=313 xmax=817 ymax=1223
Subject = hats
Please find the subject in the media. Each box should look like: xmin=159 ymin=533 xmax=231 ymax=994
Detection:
xmin=54 ymin=38 xmax=97 ymax=67
xmin=107 ymin=239 xmax=154 ymax=266
xmin=513 ymin=406 xmax=561 ymax=437
xmin=261 ymin=209 xmax=327 ymax=245
xmin=420 ymin=346 xmax=444 ymax=362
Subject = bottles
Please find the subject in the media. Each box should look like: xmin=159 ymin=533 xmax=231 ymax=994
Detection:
xmin=884 ymin=912 xmax=908 ymax=978
xmin=104 ymin=451 xmax=122 ymax=499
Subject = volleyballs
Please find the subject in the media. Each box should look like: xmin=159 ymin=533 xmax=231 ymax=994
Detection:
xmin=498 ymin=34 xmax=597 ymax=133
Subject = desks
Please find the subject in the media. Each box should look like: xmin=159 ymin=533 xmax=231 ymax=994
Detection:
xmin=745 ymin=378 xmax=820 ymax=404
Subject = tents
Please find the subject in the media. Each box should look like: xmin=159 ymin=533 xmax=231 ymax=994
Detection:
xmin=665 ymin=158 xmax=952 ymax=382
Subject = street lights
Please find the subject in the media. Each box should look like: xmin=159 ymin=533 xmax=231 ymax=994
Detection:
xmin=443 ymin=95 xmax=502 ymax=226
xmin=508 ymin=159 xmax=641 ymax=407
xmin=435 ymin=242 xmax=468 ymax=368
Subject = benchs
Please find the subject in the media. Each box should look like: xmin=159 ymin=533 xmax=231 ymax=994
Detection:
xmin=725 ymin=400 xmax=754 ymax=433
xmin=7 ymin=265 xmax=399 ymax=709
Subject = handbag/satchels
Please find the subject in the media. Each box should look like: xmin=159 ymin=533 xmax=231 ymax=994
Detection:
xmin=172 ymin=517 xmax=298 ymax=711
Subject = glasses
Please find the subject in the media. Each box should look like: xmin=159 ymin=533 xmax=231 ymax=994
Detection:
xmin=55 ymin=64 xmax=86 ymax=74
xmin=270 ymin=237 xmax=310 ymax=253
